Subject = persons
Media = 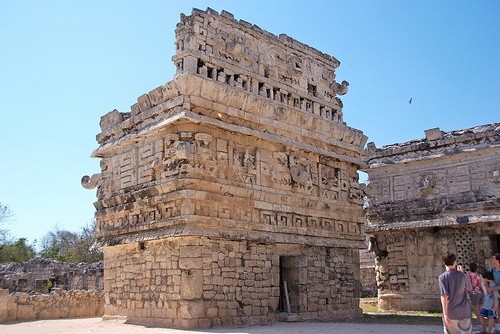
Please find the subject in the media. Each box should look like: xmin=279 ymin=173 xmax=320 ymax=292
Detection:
xmin=438 ymin=251 xmax=473 ymax=334
xmin=466 ymin=262 xmax=487 ymax=334
xmin=475 ymin=253 xmax=500 ymax=334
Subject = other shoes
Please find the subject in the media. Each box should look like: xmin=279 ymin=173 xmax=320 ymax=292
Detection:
xmin=472 ymin=324 xmax=482 ymax=331
xmin=476 ymin=331 xmax=487 ymax=334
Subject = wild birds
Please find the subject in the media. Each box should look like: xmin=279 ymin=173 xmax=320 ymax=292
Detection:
xmin=407 ymin=97 xmax=413 ymax=104
